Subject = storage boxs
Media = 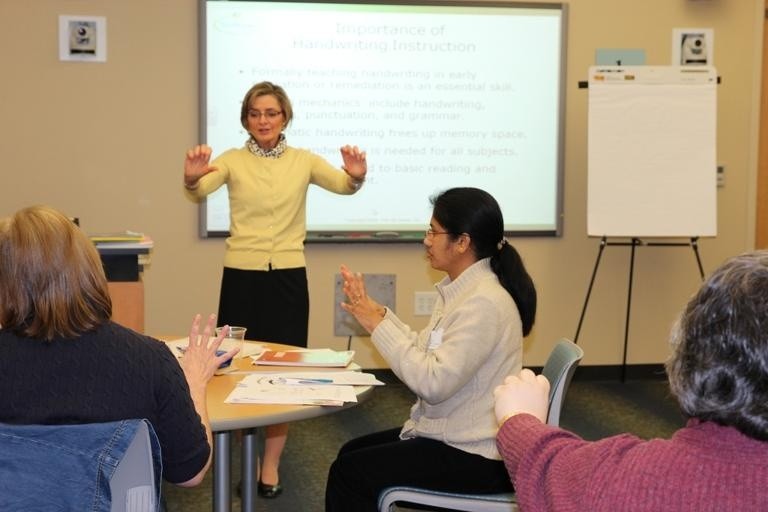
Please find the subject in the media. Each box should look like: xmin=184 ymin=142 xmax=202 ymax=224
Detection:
xmin=101 ymin=255 xmax=138 ymax=279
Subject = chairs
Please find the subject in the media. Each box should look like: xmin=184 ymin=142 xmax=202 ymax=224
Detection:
xmin=379 ymin=339 xmax=584 ymax=512
xmin=0 ymin=419 xmax=160 ymax=511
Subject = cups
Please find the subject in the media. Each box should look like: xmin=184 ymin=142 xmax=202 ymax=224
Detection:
xmin=215 ymin=325 xmax=248 ymax=373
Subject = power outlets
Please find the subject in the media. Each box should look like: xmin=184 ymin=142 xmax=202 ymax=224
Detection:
xmin=414 ymin=292 xmax=438 ymax=314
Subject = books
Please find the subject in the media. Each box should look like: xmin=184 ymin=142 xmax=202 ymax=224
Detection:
xmin=88 ymin=231 xmax=154 ymax=270
xmin=252 ymin=348 xmax=355 ymax=368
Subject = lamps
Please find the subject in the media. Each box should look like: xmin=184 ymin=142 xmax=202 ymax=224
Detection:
xmin=672 ymin=27 xmax=714 ymax=68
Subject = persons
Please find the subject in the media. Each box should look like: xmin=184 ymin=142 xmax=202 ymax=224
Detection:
xmin=326 ymin=189 xmax=523 ymax=512
xmin=495 ymin=250 xmax=767 ymax=512
xmin=182 ymin=82 xmax=367 ymax=499
xmin=1 ymin=207 xmax=240 ymax=488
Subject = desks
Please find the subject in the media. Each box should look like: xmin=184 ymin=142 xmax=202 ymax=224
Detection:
xmin=163 ymin=338 xmax=376 ymax=512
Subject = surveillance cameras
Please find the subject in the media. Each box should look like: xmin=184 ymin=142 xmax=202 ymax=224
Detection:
xmin=68 ymin=26 xmax=94 ymax=53
xmin=682 ymin=36 xmax=707 ymax=64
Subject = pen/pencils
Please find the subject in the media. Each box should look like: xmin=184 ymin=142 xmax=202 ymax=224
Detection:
xmin=176 ymin=346 xmax=187 ymax=351
xmin=298 ymin=378 xmax=334 ymax=384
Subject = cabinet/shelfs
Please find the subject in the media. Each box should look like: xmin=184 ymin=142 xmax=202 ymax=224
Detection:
xmin=573 ymin=237 xmax=714 ymax=386
xmin=99 ymin=281 xmax=144 ymax=333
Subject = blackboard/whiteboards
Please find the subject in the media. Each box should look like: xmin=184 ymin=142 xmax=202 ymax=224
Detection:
xmin=586 ymin=66 xmax=717 ymax=237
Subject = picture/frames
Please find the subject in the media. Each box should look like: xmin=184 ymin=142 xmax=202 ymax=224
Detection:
xmin=59 ymin=15 xmax=107 ymax=63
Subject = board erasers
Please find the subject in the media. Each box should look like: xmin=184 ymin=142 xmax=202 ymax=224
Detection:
xmin=375 ymin=231 xmax=403 ymax=239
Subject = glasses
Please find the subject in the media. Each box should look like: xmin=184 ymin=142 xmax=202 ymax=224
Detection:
xmin=426 ymin=229 xmax=460 ymax=240
xmin=248 ymin=111 xmax=283 ymax=119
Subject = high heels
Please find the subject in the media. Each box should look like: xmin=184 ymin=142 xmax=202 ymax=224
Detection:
xmin=259 ymin=465 xmax=283 ymax=498
xmin=237 ymin=463 xmax=260 ymax=497
